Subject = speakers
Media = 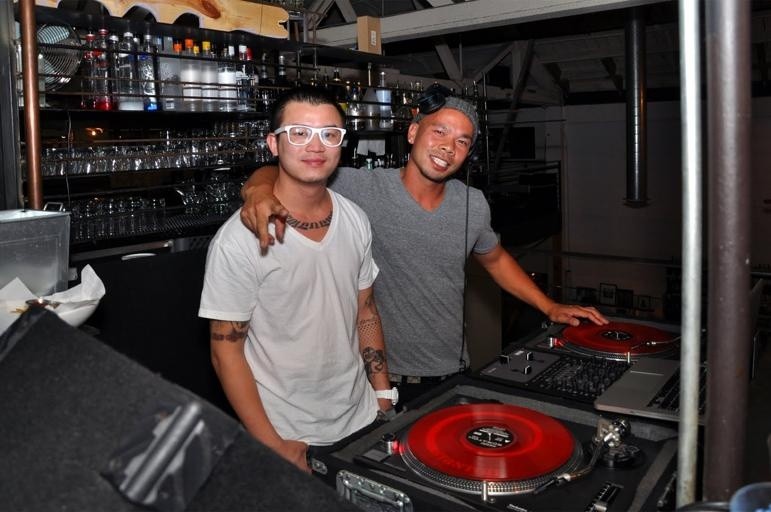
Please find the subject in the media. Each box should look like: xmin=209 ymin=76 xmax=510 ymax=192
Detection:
xmin=0 ymin=299 xmax=368 ymax=512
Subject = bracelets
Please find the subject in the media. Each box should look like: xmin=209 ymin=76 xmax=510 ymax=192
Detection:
xmin=374 ymin=386 xmax=400 ymax=406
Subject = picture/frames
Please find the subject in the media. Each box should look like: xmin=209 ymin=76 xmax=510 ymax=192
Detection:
xmin=599 ymin=283 xmax=617 ymax=306
xmin=638 ymin=295 xmax=651 ymax=311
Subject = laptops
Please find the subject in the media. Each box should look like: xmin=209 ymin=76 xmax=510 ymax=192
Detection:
xmin=594 ymin=277 xmax=763 ymax=425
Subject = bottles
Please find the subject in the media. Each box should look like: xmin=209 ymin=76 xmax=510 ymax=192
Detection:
xmin=77 ymin=29 xmax=480 ymax=130
xmin=349 ymin=148 xmax=411 ymax=171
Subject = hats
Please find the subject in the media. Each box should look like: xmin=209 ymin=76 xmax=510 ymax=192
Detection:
xmin=411 ymin=97 xmax=480 ymax=148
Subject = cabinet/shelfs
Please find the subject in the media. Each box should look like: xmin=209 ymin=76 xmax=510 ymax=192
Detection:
xmin=16 ymin=43 xmax=565 ymax=254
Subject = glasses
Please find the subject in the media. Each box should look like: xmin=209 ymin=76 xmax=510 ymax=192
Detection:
xmin=274 ymin=124 xmax=348 ymax=148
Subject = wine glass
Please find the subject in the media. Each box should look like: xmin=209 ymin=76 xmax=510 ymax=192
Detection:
xmin=31 ymin=116 xmax=273 ymax=242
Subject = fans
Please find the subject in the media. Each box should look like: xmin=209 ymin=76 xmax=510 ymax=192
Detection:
xmin=16 ymin=17 xmax=83 ymax=94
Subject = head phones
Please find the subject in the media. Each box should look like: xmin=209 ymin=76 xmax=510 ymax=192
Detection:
xmin=421 ymin=83 xmax=480 ymax=165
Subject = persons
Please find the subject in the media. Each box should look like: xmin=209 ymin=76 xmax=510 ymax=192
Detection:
xmin=197 ymin=85 xmax=397 ymax=473
xmin=241 ymin=93 xmax=610 ymax=413
